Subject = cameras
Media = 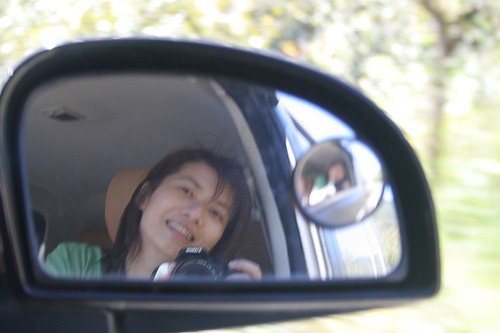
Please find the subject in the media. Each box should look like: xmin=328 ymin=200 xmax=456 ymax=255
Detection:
xmin=148 ymin=247 xmax=231 ymax=282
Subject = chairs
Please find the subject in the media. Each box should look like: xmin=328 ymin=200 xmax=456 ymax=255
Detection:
xmin=103 ymin=169 xmax=267 ymax=278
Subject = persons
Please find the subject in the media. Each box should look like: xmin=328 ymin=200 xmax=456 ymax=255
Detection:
xmin=42 ymin=145 xmax=263 ymax=284
xmin=310 ymin=161 xmax=349 ymax=201
